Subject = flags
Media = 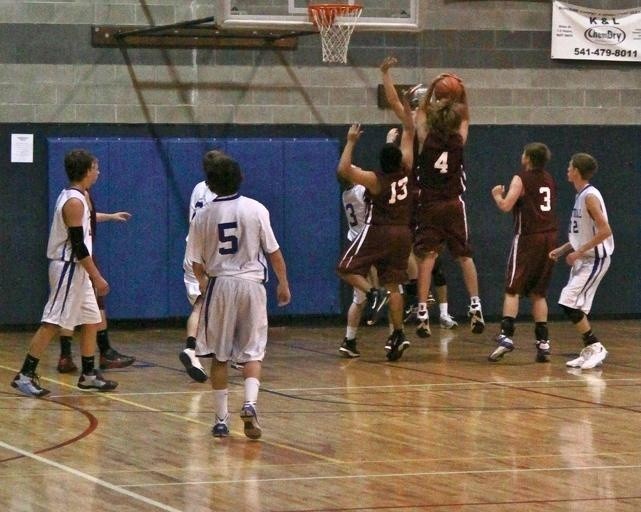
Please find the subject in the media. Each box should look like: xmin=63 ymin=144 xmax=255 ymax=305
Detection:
xmin=550 ymin=1 xmax=641 ymax=63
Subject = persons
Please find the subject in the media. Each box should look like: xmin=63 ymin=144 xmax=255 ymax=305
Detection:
xmin=177 ymin=149 xmax=246 ymax=382
xmin=10 ymin=147 xmax=116 ymax=398
xmin=55 ymin=153 xmax=134 ymax=374
xmin=186 ymin=155 xmax=291 ymax=440
xmin=486 ymin=141 xmax=559 ymax=363
xmin=547 ymin=152 xmax=614 ymax=370
xmin=334 ymin=58 xmax=488 ymax=360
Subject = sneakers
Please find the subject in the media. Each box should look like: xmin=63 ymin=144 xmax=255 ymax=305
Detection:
xmin=179 ymin=348 xmax=208 ymax=383
xmin=212 ymin=409 xmax=232 ymax=439
xmin=488 ymin=334 xmax=515 ymax=362
xmin=77 ymin=372 xmax=118 ymax=390
xmin=566 ymin=341 xmax=608 ymax=370
xmin=230 ymin=362 xmax=245 ymax=369
xmin=340 ymin=336 xmax=361 ymax=360
xmin=99 ymin=351 xmax=137 ymax=370
xmin=240 ymin=401 xmax=262 ymax=440
xmin=10 ymin=371 xmax=52 ymax=398
xmin=57 ymin=354 xmax=77 ymax=374
xmin=468 ymin=303 xmax=485 ymax=334
xmin=535 ymin=339 xmax=551 ymax=364
xmin=366 ymin=287 xmax=459 ymax=337
xmin=384 ymin=332 xmax=411 ymax=362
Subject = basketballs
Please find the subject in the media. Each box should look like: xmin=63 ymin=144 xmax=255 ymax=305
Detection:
xmin=434 ymin=76 xmax=462 ymax=101
